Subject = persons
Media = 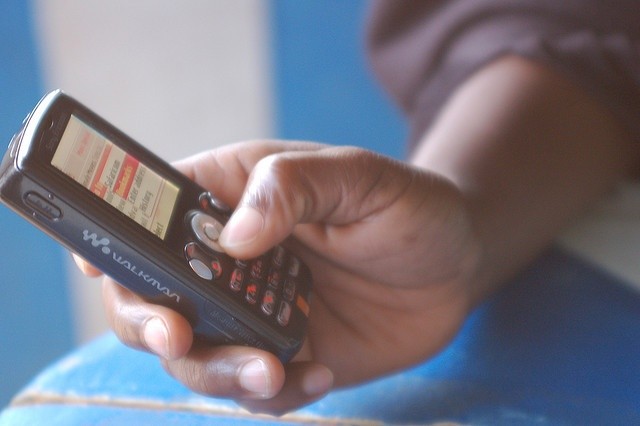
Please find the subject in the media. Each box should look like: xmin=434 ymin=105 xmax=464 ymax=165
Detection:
xmin=71 ymin=0 xmax=640 ymax=418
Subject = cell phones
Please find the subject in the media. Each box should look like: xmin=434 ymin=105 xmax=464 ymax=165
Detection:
xmin=0 ymin=88 xmax=313 ymax=366
